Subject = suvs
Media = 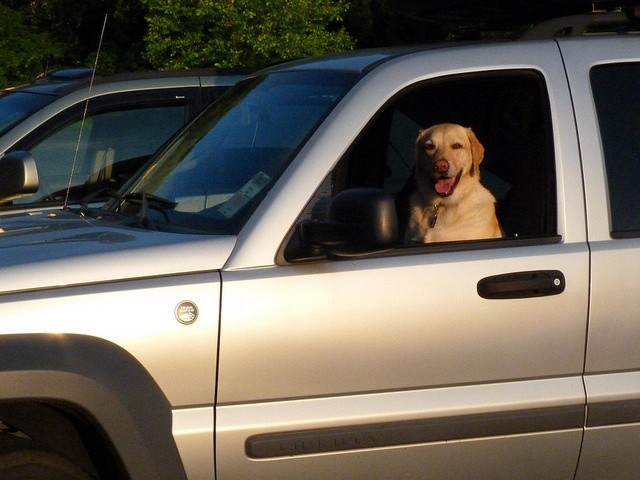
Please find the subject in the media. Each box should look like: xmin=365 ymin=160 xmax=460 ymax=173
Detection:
xmin=0 ymin=8 xmax=640 ymax=479
xmin=0 ymin=68 xmax=245 ymax=216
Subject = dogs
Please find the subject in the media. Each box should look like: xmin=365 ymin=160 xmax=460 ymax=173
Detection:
xmin=403 ymin=122 xmax=504 ymax=243
